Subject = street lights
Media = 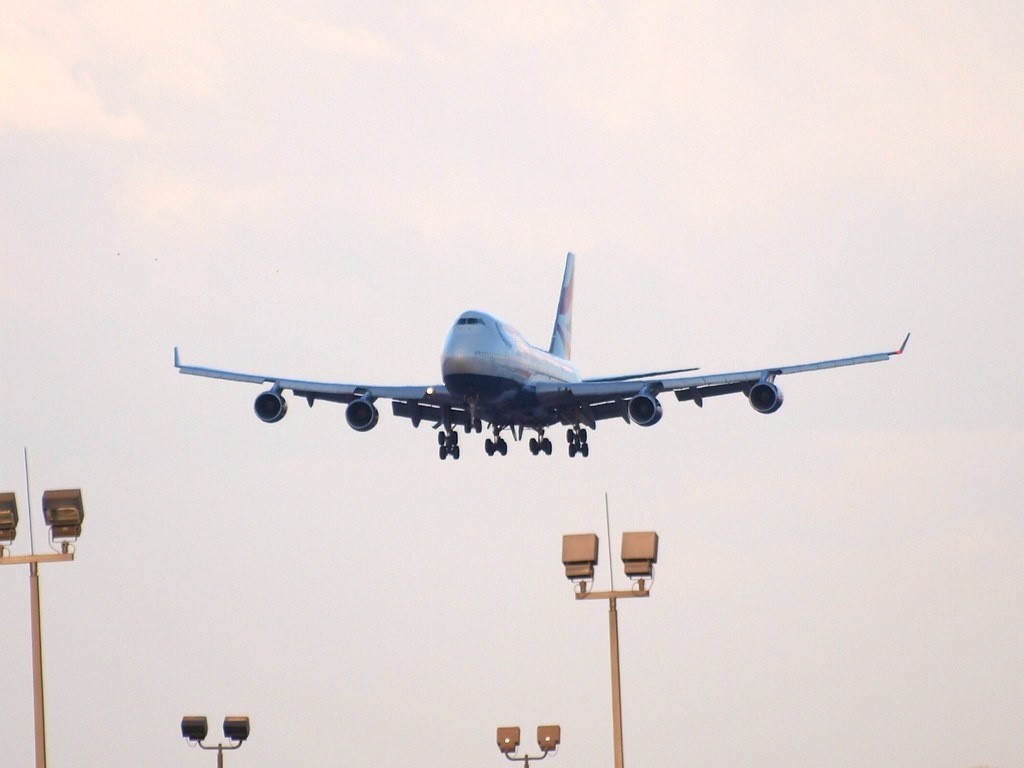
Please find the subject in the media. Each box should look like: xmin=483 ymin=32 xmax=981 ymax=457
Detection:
xmin=0 ymin=442 xmax=86 ymax=768
xmin=561 ymin=492 xmax=660 ymax=768
xmin=180 ymin=716 xmax=252 ymax=768
xmin=497 ymin=725 xmax=561 ymax=768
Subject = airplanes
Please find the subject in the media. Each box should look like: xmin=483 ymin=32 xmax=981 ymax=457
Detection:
xmin=166 ymin=251 xmax=914 ymax=460
